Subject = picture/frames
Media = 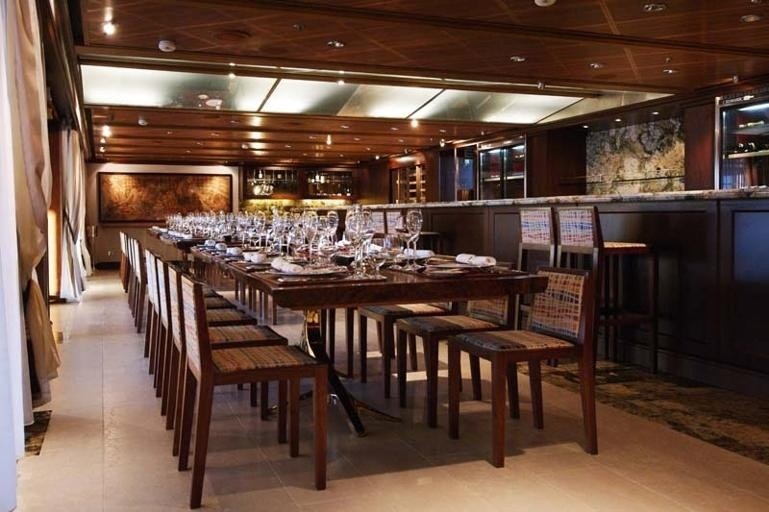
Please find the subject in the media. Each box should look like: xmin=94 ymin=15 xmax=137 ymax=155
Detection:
xmin=98 ymin=172 xmax=233 ymax=224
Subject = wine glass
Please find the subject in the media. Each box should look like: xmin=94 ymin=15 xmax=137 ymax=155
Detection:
xmin=166 ymin=208 xmax=423 ymax=279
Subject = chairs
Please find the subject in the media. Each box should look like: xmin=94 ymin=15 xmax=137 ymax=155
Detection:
xmin=447 ymin=265 xmax=599 ymax=468
xmin=395 ymin=261 xmax=521 ymax=429
xmin=555 ymin=205 xmax=660 ymax=382
xmin=517 ymin=206 xmax=611 ymax=365
xmin=120 ymin=228 xmax=332 ymax=509
xmin=357 ymin=254 xmax=463 ymax=398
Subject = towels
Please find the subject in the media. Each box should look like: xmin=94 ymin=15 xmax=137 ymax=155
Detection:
xmin=455 ymin=252 xmax=496 ymax=266
xmin=404 ymin=248 xmax=435 ymax=261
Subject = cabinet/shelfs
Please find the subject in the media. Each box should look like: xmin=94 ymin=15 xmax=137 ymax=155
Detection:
xmin=474 ymin=135 xmax=530 ymax=200
xmin=713 ymin=88 xmax=769 ymax=191
xmin=390 ymin=148 xmax=439 ymax=205
